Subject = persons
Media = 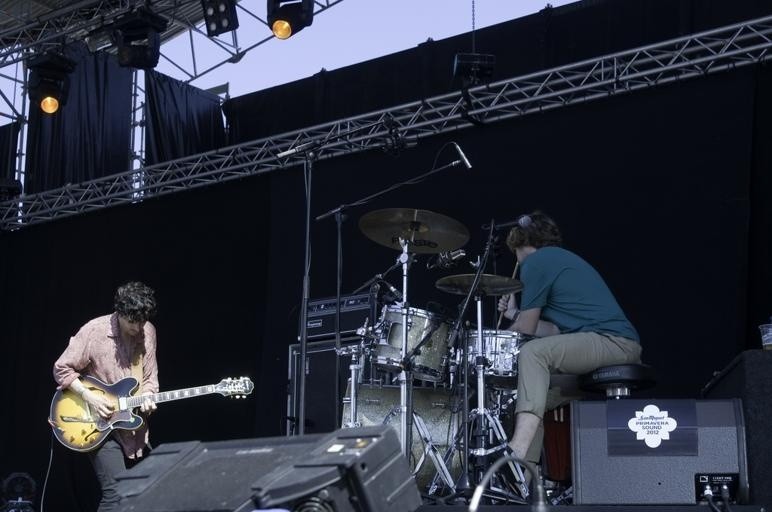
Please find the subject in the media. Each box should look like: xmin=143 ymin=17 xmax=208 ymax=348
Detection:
xmin=489 ymin=208 xmax=643 ymax=499
xmin=51 ymin=281 xmax=161 ymax=512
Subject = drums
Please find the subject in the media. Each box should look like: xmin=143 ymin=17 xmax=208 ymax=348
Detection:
xmin=371 ymin=303 xmax=458 ymax=383
xmin=458 ymin=329 xmax=529 ymax=377
xmin=485 ymin=378 xmax=516 ymax=441
xmin=341 ymin=380 xmax=474 ymax=495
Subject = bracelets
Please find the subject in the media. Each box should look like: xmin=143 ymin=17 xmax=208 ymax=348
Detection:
xmin=511 ymin=309 xmax=520 ymax=322
xmin=78 ymin=387 xmax=87 ymax=398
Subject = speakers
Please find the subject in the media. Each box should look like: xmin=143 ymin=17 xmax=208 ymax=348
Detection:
xmin=570 ymin=399 xmax=749 ymax=506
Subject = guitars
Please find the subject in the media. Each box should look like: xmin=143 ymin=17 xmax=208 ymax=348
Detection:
xmin=49 ymin=375 xmax=255 ymax=452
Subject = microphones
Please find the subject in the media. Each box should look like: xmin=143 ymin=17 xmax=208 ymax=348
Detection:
xmin=455 ymin=144 xmax=472 ymax=168
xmin=494 ymin=216 xmax=531 ymax=228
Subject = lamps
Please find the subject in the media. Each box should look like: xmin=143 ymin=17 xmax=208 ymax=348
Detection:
xmin=27 ymin=0 xmax=313 ymax=113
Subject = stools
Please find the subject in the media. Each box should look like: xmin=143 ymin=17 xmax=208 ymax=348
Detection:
xmin=553 ymin=364 xmax=655 ymax=505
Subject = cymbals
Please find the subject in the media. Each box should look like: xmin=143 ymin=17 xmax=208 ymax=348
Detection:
xmin=436 ymin=272 xmax=524 ymax=296
xmin=357 ymin=207 xmax=471 ymax=254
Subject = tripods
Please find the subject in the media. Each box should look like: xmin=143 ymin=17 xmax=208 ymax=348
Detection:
xmin=426 ymin=293 xmax=572 ymax=506
xmin=381 ymin=238 xmax=470 ymax=505
